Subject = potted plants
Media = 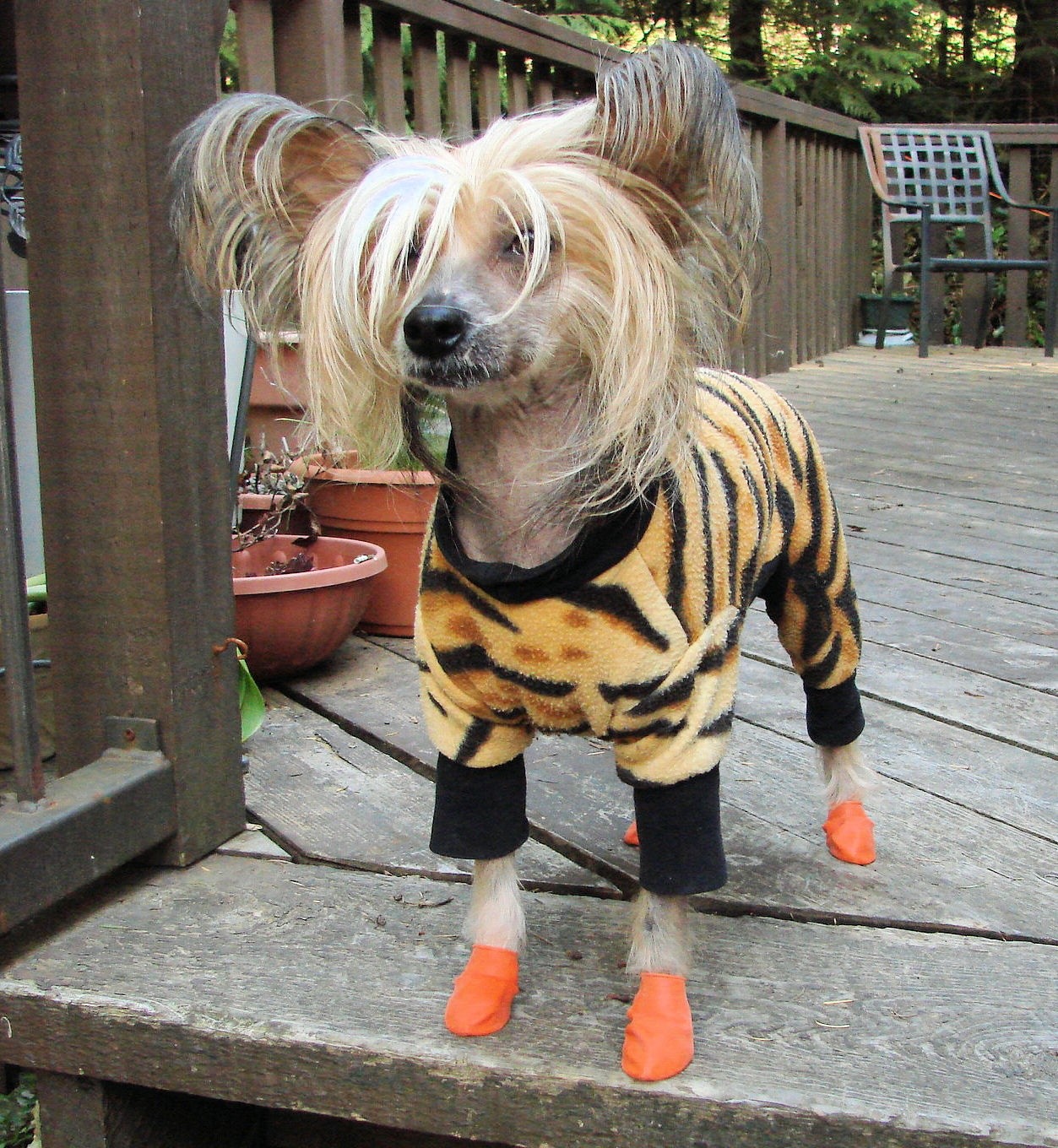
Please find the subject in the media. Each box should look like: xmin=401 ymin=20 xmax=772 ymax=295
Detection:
xmin=0 ymin=572 xmax=268 ymax=771
xmin=231 ymin=433 xmax=310 ymax=532
xmin=859 ymin=274 xmax=918 ymax=328
xmin=296 ymin=392 xmax=453 ymax=636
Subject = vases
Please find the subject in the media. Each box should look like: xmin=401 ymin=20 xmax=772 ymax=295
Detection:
xmin=229 ymin=530 xmax=385 ymax=677
xmin=245 ymin=325 xmax=358 ymax=458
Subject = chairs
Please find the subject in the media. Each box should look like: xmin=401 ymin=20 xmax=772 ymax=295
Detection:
xmin=857 ymin=125 xmax=1058 ymax=360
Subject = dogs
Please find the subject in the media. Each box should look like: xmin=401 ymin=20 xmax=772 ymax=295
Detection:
xmin=170 ymin=38 xmax=876 ymax=1080
